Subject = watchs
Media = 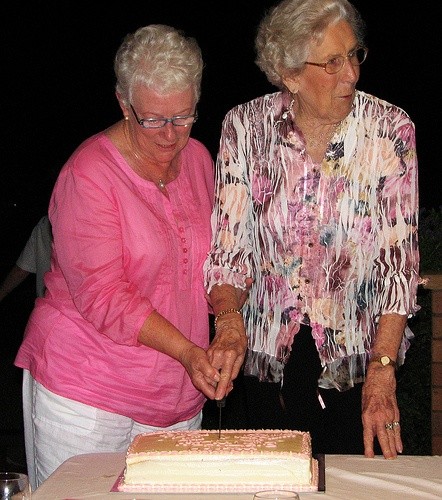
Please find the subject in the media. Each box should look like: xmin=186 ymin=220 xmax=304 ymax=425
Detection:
xmin=371 ymin=355 xmax=396 ymax=367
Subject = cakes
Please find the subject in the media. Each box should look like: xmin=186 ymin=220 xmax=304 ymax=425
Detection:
xmin=117 ymin=429 xmax=319 ymax=494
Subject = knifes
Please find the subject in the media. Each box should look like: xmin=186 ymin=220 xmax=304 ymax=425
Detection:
xmin=215 ymin=368 xmax=226 ymax=430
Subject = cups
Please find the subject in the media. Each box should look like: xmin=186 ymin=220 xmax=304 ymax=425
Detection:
xmin=252 ymin=490 xmax=300 ymax=500
xmin=0 ymin=472 xmax=32 ymax=500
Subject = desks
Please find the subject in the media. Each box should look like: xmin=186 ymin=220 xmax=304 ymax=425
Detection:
xmin=34 ymin=451 xmax=442 ymax=500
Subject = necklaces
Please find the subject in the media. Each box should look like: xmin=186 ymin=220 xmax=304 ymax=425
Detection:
xmin=159 ymin=179 xmax=164 ymax=188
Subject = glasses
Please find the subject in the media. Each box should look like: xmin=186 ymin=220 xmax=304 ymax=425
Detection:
xmin=305 ymin=43 xmax=368 ymax=74
xmin=129 ymin=102 xmax=199 ymax=129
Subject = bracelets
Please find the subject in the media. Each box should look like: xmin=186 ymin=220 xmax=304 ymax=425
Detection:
xmin=214 ymin=308 xmax=241 ymax=330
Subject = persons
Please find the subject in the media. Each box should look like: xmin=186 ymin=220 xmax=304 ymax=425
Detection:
xmin=202 ymin=0 xmax=420 ymax=458
xmin=14 ymin=24 xmax=254 ymax=492
xmin=0 ymin=215 xmax=53 ymax=301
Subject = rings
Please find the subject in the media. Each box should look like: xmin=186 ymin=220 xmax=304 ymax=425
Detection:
xmin=385 ymin=421 xmax=399 ymax=430
